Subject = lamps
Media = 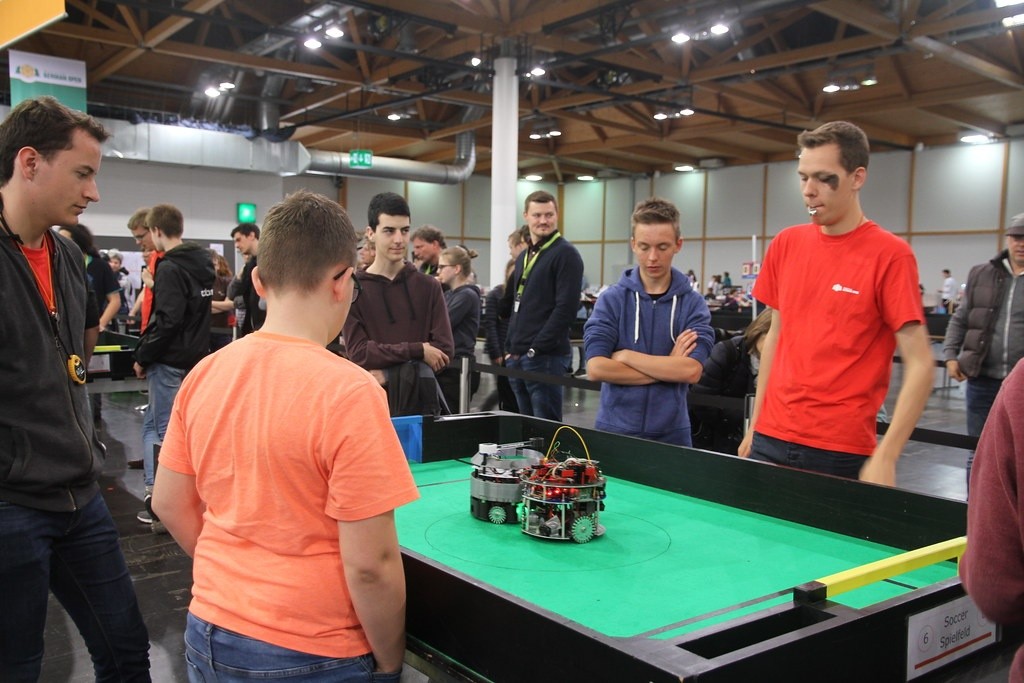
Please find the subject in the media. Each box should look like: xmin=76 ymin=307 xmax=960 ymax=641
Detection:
xmin=302 ymin=13 xmax=347 ymax=52
xmin=822 ymin=56 xmax=878 ymax=92
xmin=200 ymin=75 xmax=239 ymax=103
xmin=526 ymin=121 xmax=562 ymax=143
xmin=670 ymin=16 xmax=729 ymax=43
xmin=651 ymin=100 xmax=699 ymax=123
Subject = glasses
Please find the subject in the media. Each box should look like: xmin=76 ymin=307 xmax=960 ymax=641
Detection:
xmin=133 ymin=232 xmax=148 ymax=240
xmin=333 ymin=267 xmax=362 ymax=304
xmin=436 ymin=265 xmax=456 ymax=269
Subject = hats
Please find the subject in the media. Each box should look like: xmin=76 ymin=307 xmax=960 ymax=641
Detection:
xmin=1005 ymin=213 xmax=1024 ymax=235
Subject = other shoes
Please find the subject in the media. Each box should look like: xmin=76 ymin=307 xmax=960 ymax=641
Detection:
xmin=137 ymin=511 xmax=153 ymax=523
xmin=144 ymin=492 xmax=167 ymax=535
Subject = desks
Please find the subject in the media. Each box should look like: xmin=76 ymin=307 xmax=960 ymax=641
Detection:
xmin=149 ymin=409 xmax=1012 ymax=683
xmin=709 ymin=303 xmax=959 ymax=399
xmin=85 ymin=328 xmax=148 ymax=430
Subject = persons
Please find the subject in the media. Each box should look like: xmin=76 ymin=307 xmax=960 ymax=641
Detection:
xmin=738 ymin=120 xmax=935 ymax=487
xmin=686 ymin=270 xmax=761 ymax=314
xmin=940 ymin=213 xmax=1023 ymax=500
xmin=959 ymin=354 xmax=1024 ymax=683
xmin=0 ymin=94 xmax=150 ymax=683
xmin=59 ymin=190 xmax=584 ymax=524
xmin=939 ymin=271 xmax=965 ymax=316
xmin=584 ymin=198 xmax=716 ymax=447
xmin=150 ymin=188 xmax=421 ymax=682
xmin=687 ymin=307 xmax=772 ymax=457
